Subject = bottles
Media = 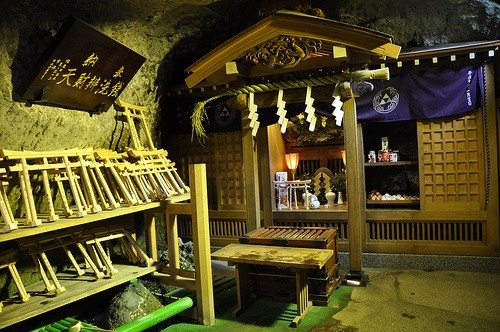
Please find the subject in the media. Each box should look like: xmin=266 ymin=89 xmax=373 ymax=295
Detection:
xmin=325 ymin=190 xmax=336 ymax=206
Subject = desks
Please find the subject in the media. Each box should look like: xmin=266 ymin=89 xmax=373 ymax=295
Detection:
xmin=211 ymin=243 xmax=334 ymax=328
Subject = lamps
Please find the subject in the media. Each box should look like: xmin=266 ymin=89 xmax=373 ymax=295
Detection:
xmin=285 ymin=153 xmax=300 ymax=209
xmin=339 ymin=148 xmax=348 ymax=206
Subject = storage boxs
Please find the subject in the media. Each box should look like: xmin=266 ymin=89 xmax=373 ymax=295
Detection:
xmin=239 ymin=224 xmax=342 ymax=308
